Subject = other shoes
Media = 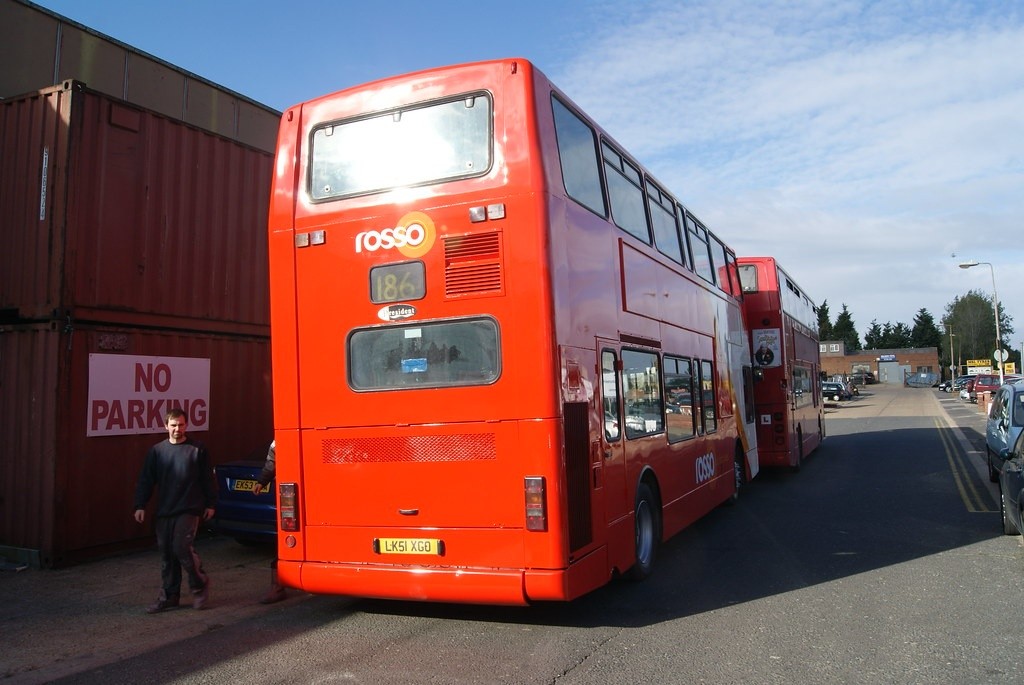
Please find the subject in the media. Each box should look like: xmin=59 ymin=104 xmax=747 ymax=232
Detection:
xmin=192 ymin=577 xmax=210 ymax=610
xmin=145 ymin=590 xmax=179 ymax=613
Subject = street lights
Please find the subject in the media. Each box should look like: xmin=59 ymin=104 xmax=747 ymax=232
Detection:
xmin=936 ymin=324 xmax=955 ymax=393
xmin=959 ymin=258 xmax=1006 ymax=387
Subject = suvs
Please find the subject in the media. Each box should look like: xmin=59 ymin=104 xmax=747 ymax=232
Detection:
xmin=605 ymin=411 xmax=619 ymax=440
xmin=953 ymin=375 xmax=979 ymax=391
xmin=984 ymin=380 xmax=1024 ymax=483
xmin=966 ymin=375 xmax=1023 ymax=404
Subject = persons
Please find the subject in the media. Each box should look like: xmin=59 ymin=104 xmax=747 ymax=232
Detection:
xmin=252 ymin=438 xmax=289 ymax=606
xmin=134 ymin=408 xmax=216 ymax=613
xmin=754 ymin=341 xmax=775 ymax=366
xmin=847 ymin=380 xmax=854 ymax=400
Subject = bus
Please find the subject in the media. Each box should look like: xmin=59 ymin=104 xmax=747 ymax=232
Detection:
xmin=266 ymin=56 xmax=769 ymax=609
xmin=694 ymin=255 xmax=829 ymax=471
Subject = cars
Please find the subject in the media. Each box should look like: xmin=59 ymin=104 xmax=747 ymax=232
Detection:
xmin=938 ymin=379 xmax=956 ymax=393
xmin=960 ymin=387 xmax=978 ymax=404
xmin=822 ymin=370 xmax=876 ymax=402
xmin=211 ymin=449 xmax=277 ymax=547
xmin=998 ymin=428 xmax=1024 ymax=538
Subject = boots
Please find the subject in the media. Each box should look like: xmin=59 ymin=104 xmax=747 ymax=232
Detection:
xmin=259 ymin=569 xmax=287 ymax=604
